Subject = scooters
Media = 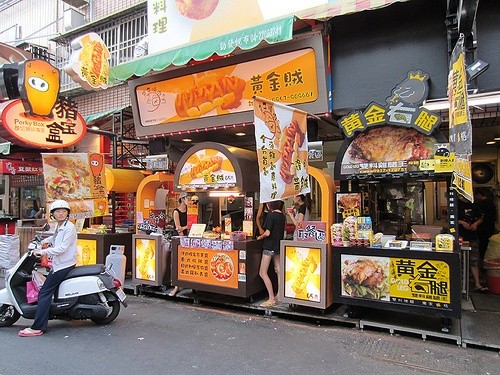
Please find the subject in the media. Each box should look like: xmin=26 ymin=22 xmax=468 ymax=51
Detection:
xmin=0 ymin=235 xmax=128 ymax=326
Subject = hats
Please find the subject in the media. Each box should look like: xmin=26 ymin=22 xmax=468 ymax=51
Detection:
xmin=178 ymin=191 xmax=189 ymax=199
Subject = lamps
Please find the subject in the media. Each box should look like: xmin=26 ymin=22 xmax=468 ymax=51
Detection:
xmin=464 ymin=59 xmax=490 ymax=82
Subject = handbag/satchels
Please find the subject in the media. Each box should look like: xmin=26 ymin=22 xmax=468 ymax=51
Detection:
xmin=25 ymin=269 xmax=46 ymax=305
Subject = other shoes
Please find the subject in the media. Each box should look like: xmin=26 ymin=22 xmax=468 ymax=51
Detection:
xmin=260 ymin=300 xmax=277 ymax=308
xmin=474 ymin=283 xmax=488 ymax=293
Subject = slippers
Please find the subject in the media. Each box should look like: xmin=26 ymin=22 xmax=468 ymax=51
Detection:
xmin=18 ymin=327 xmax=44 ymax=337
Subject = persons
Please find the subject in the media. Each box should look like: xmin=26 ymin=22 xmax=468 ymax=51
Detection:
xmin=17 ymin=199 xmax=78 ymax=337
xmin=168 ymin=192 xmax=187 ymax=297
xmin=25 ymin=205 xmax=36 ymax=219
xmin=458 ymin=186 xmax=497 ymax=293
xmin=256 ymin=194 xmax=311 ymax=308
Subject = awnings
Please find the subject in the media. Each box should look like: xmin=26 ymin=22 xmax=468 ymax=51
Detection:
xmin=108 ymin=14 xmax=310 ymax=83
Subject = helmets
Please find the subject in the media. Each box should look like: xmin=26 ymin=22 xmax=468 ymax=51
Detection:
xmin=49 ymin=200 xmax=71 ymax=222
